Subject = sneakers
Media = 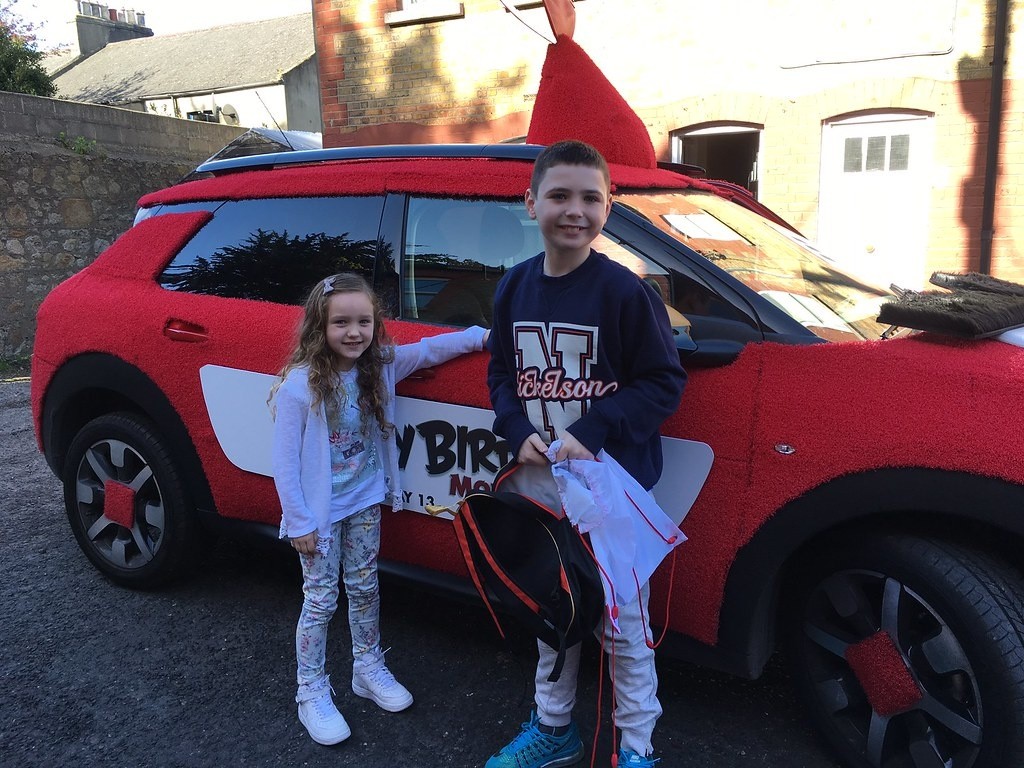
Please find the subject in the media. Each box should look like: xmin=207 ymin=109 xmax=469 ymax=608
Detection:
xmin=294 ymin=675 xmax=352 ymax=744
xmin=483 ymin=709 xmax=584 ymax=768
xmin=616 ymin=748 xmax=661 ymax=768
xmin=353 ymin=647 xmax=412 ymax=712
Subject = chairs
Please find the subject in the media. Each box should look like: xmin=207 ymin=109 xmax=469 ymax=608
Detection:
xmin=426 ymin=206 xmax=527 ymax=329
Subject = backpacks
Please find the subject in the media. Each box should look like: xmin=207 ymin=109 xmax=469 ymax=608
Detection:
xmin=426 ymin=456 xmax=607 ymax=683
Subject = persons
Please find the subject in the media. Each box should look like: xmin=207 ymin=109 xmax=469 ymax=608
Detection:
xmin=481 ymin=140 xmax=686 ymax=768
xmin=265 ymin=274 xmax=490 ymax=748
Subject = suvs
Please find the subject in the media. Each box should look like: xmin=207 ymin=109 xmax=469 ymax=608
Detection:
xmin=30 ymin=144 xmax=1024 ymax=768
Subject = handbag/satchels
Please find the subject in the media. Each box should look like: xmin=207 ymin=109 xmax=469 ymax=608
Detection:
xmin=543 ymin=441 xmax=689 ymax=648
